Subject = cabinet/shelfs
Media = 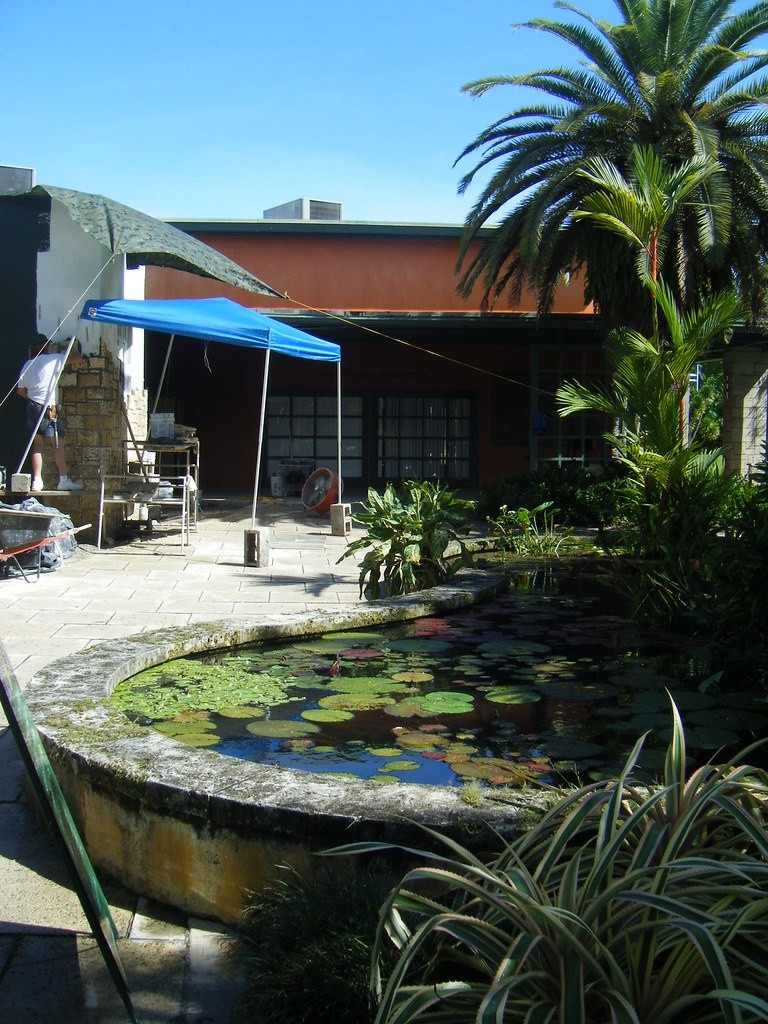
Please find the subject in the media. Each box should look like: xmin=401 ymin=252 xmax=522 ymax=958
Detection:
xmin=95 ymin=441 xmax=201 ymax=554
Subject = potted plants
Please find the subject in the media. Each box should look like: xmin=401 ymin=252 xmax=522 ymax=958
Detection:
xmin=280 ymin=460 xmax=316 ymax=491
xmin=332 ymin=480 xmax=476 ymax=602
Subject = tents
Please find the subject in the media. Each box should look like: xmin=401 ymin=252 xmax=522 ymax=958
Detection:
xmin=12 ymin=296 xmax=341 ymax=530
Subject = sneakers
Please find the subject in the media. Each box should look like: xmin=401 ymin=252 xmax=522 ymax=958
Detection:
xmin=57 ymin=478 xmax=83 ymax=492
xmin=32 ymin=481 xmax=48 ymax=491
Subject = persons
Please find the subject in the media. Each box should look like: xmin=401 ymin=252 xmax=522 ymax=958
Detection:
xmin=17 ymin=334 xmax=84 ymax=491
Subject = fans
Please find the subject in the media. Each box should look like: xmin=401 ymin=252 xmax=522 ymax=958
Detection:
xmin=300 ymin=468 xmax=344 ymax=514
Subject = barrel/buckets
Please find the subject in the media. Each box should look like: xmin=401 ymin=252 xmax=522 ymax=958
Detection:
xmin=150 ymin=413 xmax=175 ymax=443
